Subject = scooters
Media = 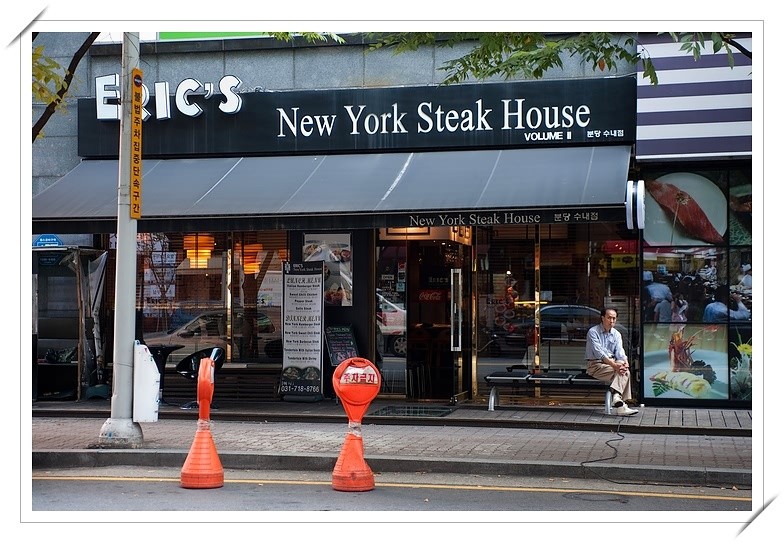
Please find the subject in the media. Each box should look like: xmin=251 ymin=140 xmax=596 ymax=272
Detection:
xmin=477 ymin=306 xmax=503 ymax=359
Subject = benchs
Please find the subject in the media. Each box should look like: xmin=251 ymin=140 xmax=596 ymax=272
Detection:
xmin=164 ymin=362 xmax=283 ymax=401
xmin=485 ymin=370 xmax=613 ymax=414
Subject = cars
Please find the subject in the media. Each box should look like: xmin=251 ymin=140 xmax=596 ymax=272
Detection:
xmin=143 ymin=306 xmax=282 ymax=368
xmin=501 ymin=304 xmax=627 ymax=348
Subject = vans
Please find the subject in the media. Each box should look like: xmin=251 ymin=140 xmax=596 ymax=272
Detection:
xmin=340 ymin=272 xmax=407 ymax=359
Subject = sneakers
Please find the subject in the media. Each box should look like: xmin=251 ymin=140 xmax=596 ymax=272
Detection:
xmin=612 ymin=396 xmax=623 ymax=408
xmin=618 ymin=404 xmax=639 ymax=416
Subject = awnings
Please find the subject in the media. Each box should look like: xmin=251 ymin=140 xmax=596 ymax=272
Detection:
xmin=32 ymin=143 xmax=632 ymax=235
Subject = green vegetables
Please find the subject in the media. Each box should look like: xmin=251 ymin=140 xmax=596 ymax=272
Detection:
xmin=652 ymin=378 xmax=670 ymax=396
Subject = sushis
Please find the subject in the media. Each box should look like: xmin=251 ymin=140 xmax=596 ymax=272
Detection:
xmin=324 ymin=290 xmax=343 ymax=305
xmin=646 ymin=181 xmax=725 ymax=243
xmin=650 ymin=369 xmax=711 ymax=397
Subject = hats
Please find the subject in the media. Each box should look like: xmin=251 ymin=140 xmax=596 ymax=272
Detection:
xmin=743 ymin=264 xmax=751 ymax=271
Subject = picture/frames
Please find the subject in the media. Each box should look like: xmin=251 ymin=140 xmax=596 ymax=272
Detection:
xmin=453 ymin=226 xmax=470 ymax=238
xmin=386 ymin=226 xmax=430 ymax=235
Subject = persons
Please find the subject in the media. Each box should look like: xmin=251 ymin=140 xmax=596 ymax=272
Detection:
xmin=643 ymin=257 xmax=752 ymax=322
xmin=585 ymin=307 xmax=639 ymax=417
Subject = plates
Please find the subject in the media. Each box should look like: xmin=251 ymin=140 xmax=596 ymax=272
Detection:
xmin=644 ymin=349 xmax=729 ymax=399
xmin=495 ymin=304 xmax=516 ymax=330
xmin=644 ymin=172 xmax=727 ymax=247
